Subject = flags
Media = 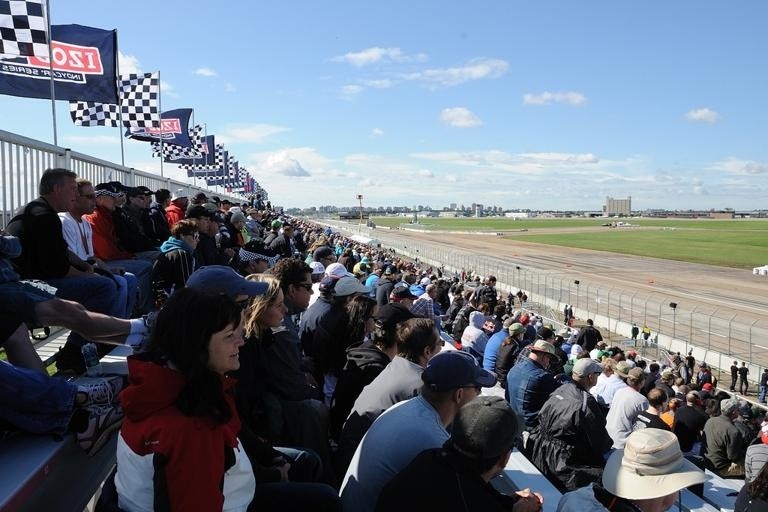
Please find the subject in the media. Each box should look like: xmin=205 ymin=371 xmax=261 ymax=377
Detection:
xmin=0 ymin=0 xmax=269 ymax=198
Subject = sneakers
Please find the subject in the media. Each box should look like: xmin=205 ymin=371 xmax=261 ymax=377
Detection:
xmin=130 ymin=308 xmax=163 ymax=355
xmin=71 ymin=374 xmax=129 ymax=458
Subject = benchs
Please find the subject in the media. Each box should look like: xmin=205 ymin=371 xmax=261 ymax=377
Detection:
xmin=432 ymin=324 xmax=746 ymax=512
xmin=1 ymin=311 xmax=149 ymax=509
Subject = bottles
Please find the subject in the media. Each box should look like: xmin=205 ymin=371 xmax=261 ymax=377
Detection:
xmin=240 ymin=226 xmax=249 ymax=244
xmin=81 ymin=343 xmax=103 ymax=378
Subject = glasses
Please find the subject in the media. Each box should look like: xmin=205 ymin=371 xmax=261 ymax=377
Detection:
xmin=183 ymin=232 xmax=200 ymax=240
xmin=298 ymin=282 xmax=312 ymax=289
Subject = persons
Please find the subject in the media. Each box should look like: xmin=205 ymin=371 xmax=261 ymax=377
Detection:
xmin=371 ymin=396 xmax=545 ymax=511
xmin=335 ymin=348 xmax=500 ymax=512
xmin=734 ymin=459 xmax=768 ymax=512
xmin=557 ymin=428 xmax=708 ymax=511
xmin=116 ymin=289 xmax=344 ymax=511
xmin=0 ymin=168 xmax=767 ymax=487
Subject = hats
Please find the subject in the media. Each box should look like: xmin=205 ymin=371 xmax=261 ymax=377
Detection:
xmin=185 ymin=202 xmax=250 ymax=223
xmin=310 ymin=251 xmax=739 ymax=499
xmin=109 ymin=182 xmax=210 ymax=201
xmin=185 ymin=265 xmax=269 ymax=299
